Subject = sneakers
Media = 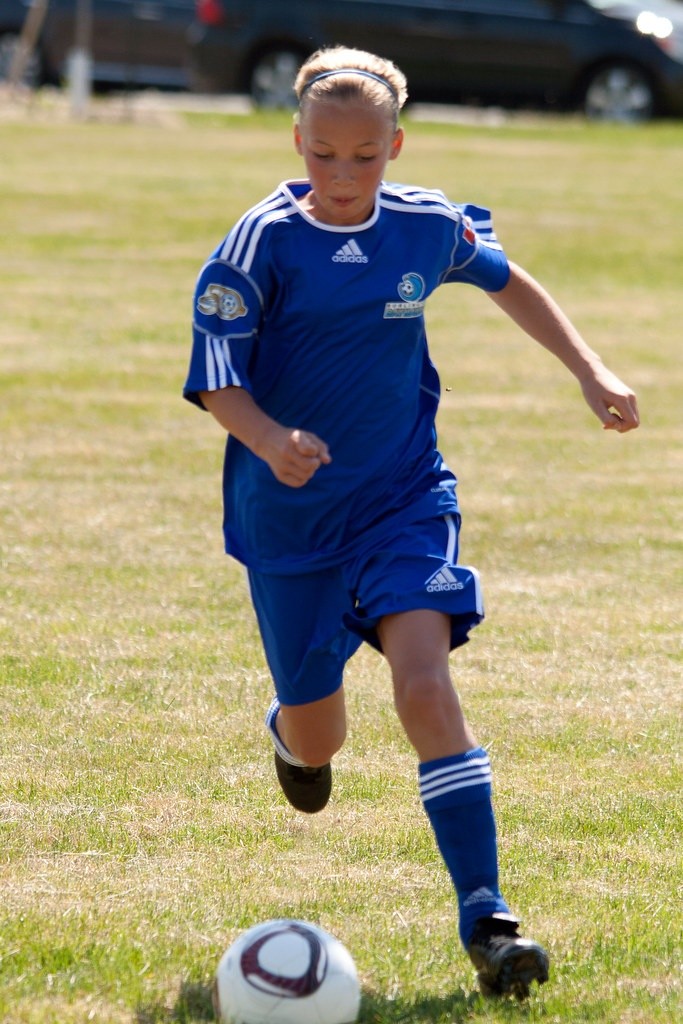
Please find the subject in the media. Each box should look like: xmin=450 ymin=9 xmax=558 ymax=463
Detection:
xmin=463 ymin=914 xmax=550 ymax=997
xmin=274 ymin=751 xmax=332 ymax=814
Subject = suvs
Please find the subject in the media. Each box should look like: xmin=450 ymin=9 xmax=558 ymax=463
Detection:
xmin=0 ymin=0 xmax=683 ymax=121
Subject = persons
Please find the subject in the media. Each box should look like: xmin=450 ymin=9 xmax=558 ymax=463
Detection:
xmin=180 ymin=43 xmax=641 ymax=1003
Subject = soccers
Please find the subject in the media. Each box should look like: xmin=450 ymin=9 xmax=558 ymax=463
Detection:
xmin=211 ymin=918 xmax=361 ymax=1024
